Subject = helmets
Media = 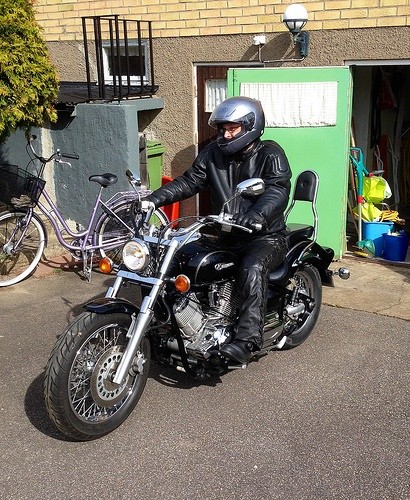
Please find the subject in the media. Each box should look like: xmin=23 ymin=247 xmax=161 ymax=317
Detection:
xmin=213 ymin=96 xmax=265 ymax=155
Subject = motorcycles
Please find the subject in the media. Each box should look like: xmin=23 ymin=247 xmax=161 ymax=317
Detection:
xmin=41 ymin=170 xmax=350 ymax=441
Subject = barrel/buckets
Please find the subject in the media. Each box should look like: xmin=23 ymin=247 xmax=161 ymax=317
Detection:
xmin=381 ymin=233 xmax=409 ymax=261
xmin=362 ymin=221 xmax=395 ymax=257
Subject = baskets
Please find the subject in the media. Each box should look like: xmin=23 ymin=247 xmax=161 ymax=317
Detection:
xmin=0 ymin=165 xmax=46 ymax=211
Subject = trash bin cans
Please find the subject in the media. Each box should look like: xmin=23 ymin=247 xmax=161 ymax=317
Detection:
xmin=139 ymin=141 xmax=163 ymax=225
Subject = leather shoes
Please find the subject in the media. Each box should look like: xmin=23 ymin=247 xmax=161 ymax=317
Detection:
xmin=219 ymin=340 xmax=252 ymax=368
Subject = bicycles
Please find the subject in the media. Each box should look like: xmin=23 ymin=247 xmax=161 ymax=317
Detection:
xmin=0 ymin=124 xmax=170 ymax=288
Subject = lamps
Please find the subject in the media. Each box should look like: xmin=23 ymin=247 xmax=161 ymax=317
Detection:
xmin=283 ymin=4 xmax=310 ymax=57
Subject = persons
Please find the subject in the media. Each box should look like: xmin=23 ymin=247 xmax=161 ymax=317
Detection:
xmin=130 ymin=96 xmax=293 ymax=365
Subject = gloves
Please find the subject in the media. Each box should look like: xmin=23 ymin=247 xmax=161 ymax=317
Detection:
xmin=129 ymin=197 xmax=151 ymax=218
xmin=232 ymin=212 xmax=257 ymax=232
